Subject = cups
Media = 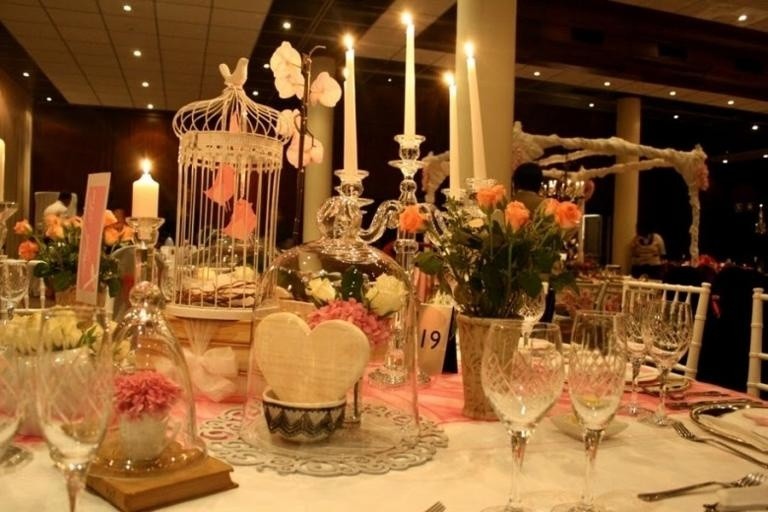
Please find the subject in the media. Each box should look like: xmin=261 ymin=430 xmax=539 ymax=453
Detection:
xmin=118 ymin=413 xmax=179 ymax=465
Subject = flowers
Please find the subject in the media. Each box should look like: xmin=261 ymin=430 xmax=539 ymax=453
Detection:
xmin=307 ymin=271 xmax=405 ymax=355
xmin=111 ymin=368 xmax=183 ymax=419
xmin=399 ymin=180 xmax=582 ymax=315
xmin=14 ymin=200 xmax=132 ymax=285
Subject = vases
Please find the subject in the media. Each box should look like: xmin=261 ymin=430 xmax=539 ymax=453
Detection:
xmin=453 ymin=309 xmax=531 ymax=421
xmin=117 ymin=410 xmax=184 ymax=460
xmin=54 ymin=284 xmax=112 ymax=334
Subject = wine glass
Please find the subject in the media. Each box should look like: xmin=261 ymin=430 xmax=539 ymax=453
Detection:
xmin=480 ymin=319 xmax=564 ymax=512
xmin=614 ymin=291 xmax=692 ymax=426
xmin=1 ymin=261 xmax=113 ymax=512
xmin=549 ymin=310 xmax=628 ymax=511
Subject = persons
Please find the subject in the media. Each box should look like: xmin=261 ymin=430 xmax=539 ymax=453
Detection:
xmin=102 ymin=207 xmax=134 ymax=246
xmin=42 ymin=189 xmax=73 ymax=244
xmin=631 ymin=223 xmax=667 ymax=267
xmin=510 ymin=163 xmax=556 ymax=323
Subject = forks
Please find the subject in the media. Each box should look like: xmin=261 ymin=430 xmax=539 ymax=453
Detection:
xmin=673 ymin=420 xmax=765 ymax=470
xmin=425 ymin=501 xmax=447 ymax=512
xmin=640 ymin=470 xmax=767 ymax=502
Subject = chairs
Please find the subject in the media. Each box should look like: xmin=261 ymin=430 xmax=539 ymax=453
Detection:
xmin=619 ymin=276 xmax=713 ymax=380
xmin=748 ymin=284 xmax=768 ymax=396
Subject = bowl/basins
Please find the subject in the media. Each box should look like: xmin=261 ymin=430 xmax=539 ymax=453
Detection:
xmin=259 ymin=393 xmax=347 ymax=446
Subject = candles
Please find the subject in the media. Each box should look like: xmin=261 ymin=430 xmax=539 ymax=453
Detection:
xmin=132 ymin=172 xmax=160 ymax=218
xmin=449 ymin=86 xmax=461 ymax=189
xmin=467 ymin=57 xmax=486 ymax=178
xmin=404 ymin=26 xmax=417 ymax=136
xmin=343 ymin=49 xmax=358 ymax=171
xmin=0 ymin=137 xmax=6 ymax=201
